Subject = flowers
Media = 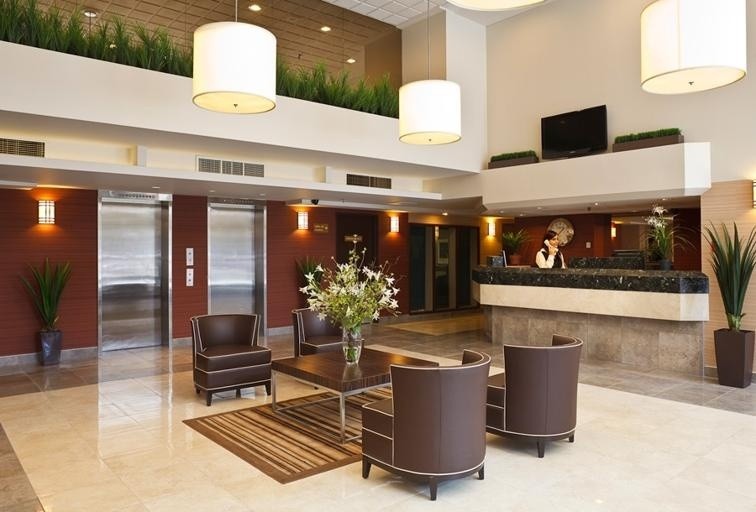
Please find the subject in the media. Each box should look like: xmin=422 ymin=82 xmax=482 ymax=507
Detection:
xmin=297 ymin=245 xmax=408 ymax=361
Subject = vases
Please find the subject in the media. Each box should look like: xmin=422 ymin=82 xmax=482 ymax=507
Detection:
xmin=342 ymin=327 xmax=362 ymax=365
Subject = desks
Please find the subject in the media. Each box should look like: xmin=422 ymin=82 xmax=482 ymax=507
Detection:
xmin=269 ymin=348 xmax=440 ymax=445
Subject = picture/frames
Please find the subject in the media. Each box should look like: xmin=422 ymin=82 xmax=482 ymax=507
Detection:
xmin=437 ymin=236 xmax=449 ymax=266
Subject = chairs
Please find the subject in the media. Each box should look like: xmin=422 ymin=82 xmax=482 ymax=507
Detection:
xmin=290 ymin=306 xmax=365 ymax=390
xmin=189 ymin=313 xmax=273 ymax=406
xmin=361 ymin=333 xmax=584 ymax=501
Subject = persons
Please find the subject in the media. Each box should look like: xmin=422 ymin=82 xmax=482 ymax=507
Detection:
xmin=534 ymin=230 xmax=566 ymax=270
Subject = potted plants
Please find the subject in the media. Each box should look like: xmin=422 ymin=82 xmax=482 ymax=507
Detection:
xmin=612 ymin=128 xmax=684 ymax=153
xmin=699 ymin=218 xmax=756 ymax=388
xmin=487 ymin=150 xmax=539 ymax=169
xmin=502 ymin=227 xmax=534 ymax=265
xmin=628 ymin=199 xmax=700 ymax=270
xmin=16 ymin=253 xmax=75 ymax=368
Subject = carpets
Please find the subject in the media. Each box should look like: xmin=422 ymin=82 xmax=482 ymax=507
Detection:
xmin=181 ymin=385 xmax=393 ymax=485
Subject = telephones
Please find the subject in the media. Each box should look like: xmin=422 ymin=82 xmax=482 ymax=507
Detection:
xmin=544 ymin=239 xmax=558 ymax=253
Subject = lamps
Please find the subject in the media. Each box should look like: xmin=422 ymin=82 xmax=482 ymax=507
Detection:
xmin=640 ymin=0 xmax=746 ymax=95
xmin=397 ymin=1 xmax=462 ymax=147
xmin=192 ymin=1 xmax=277 ymax=115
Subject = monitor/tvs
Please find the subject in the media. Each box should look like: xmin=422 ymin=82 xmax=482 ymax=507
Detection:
xmin=540 ymin=104 xmax=608 ymax=160
xmin=501 ymin=249 xmax=508 ymax=267
